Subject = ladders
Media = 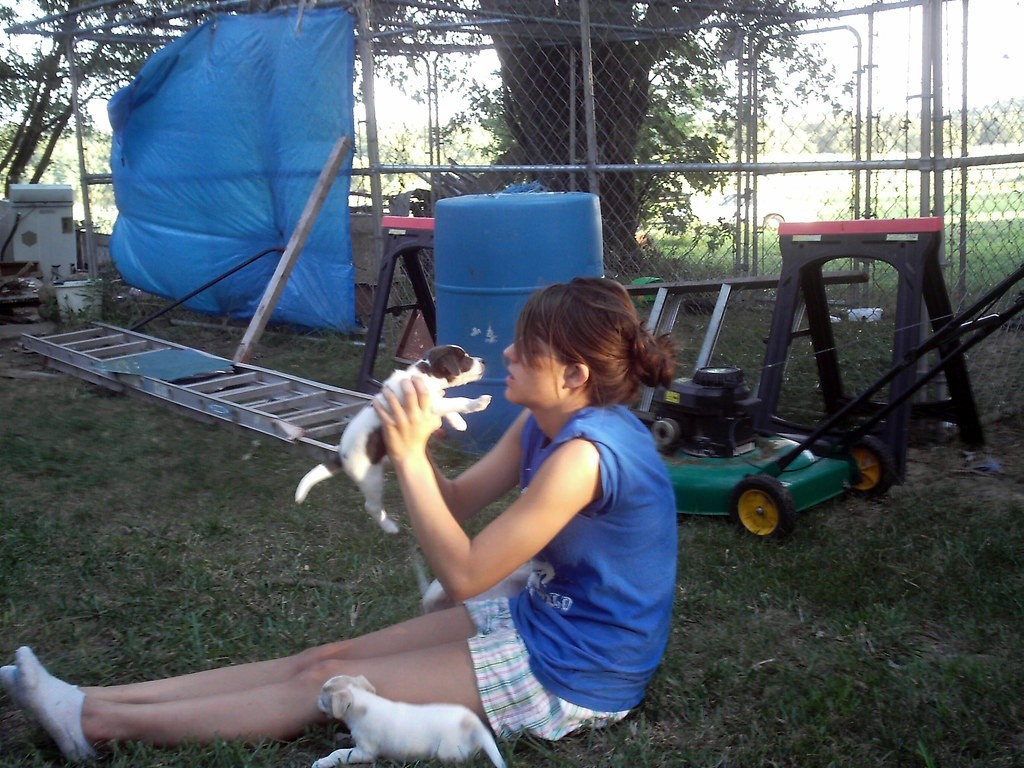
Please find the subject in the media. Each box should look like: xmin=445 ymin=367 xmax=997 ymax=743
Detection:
xmin=18 ymin=312 xmax=376 ymax=459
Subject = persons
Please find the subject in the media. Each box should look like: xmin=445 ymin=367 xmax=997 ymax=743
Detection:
xmin=0 ymin=278 xmax=678 ymax=763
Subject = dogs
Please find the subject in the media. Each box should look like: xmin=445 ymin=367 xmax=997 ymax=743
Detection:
xmin=294 ymin=343 xmax=492 ymax=535
xmin=311 ymin=673 xmax=508 ymax=768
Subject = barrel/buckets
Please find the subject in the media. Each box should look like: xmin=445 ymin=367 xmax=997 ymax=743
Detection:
xmin=432 ymin=192 xmax=604 ymax=453
xmin=53 ymin=280 xmax=103 ymax=321
